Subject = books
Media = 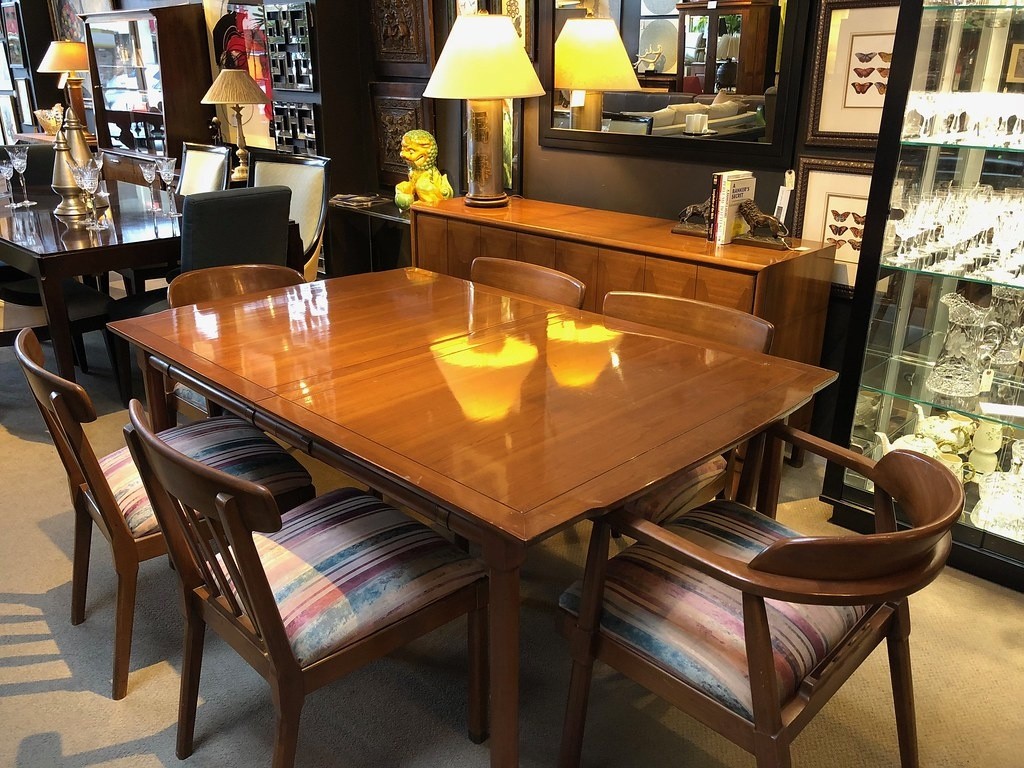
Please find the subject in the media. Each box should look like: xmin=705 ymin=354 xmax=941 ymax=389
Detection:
xmin=708 ymin=170 xmax=757 ymax=245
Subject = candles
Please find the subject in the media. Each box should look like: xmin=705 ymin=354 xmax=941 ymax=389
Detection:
xmin=686 ymin=115 xmax=695 ymax=132
xmin=700 ymin=114 xmax=709 ymax=132
xmin=693 ymin=114 xmax=701 ymax=132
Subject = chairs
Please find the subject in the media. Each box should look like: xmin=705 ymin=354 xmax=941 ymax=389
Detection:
xmin=559 ymin=419 xmax=963 ymax=768
xmin=12 ymin=327 xmax=315 ymax=700
xmin=0 ymin=143 xmax=335 ymax=408
xmin=123 ymin=397 xmax=486 ymax=768
xmin=467 ymin=255 xmax=776 ymax=530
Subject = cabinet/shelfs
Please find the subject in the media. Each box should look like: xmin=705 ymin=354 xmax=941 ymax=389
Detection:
xmin=410 ymin=194 xmax=836 ymax=468
xmin=0 ymin=0 xmax=67 ymax=145
xmin=690 ymin=62 xmax=705 ymax=93
xmin=675 ymin=0 xmax=782 ymax=94
xmin=76 ymin=2 xmax=217 ymax=193
xmin=818 ymin=0 xmax=1024 ymax=595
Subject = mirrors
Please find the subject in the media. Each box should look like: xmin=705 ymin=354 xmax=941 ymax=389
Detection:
xmin=539 ymin=0 xmax=813 ymax=172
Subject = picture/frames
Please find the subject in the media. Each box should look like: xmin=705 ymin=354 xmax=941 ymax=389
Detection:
xmin=787 ymin=155 xmax=923 ymax=308
xmin=800 ymin=0 xmax=947 ymax=154
xmin=1002 ymin=40 xmax=1024 ymax=89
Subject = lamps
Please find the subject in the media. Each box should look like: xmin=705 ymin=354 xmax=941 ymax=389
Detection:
xmin=36 ymin=38 xmax=96 ymax=140
xmin=430 ymin=277 xmax=622 ymax=421
xmin=423 ymin=8 xmax=546 ymax=208
xmin=554 ymin=15 xmax=642 ymax=132
xmin=201 ymin=69 xmax=271 ymax=178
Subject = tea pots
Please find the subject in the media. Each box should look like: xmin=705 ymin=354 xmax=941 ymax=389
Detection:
xmin=875 ymin=431 xmax=959 ymax=459
xmin=913 ymin=403 xmax=973 ymax=455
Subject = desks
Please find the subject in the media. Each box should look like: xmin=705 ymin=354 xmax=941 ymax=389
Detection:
xmin=15 ymin=133 xmax=96 ymax=146
xmin=637 ymin=75 xmax=675 ymax=93
xmin=105 ymin=267 xmax=838 ymax=768
xmin=0 ymin=175 xmax=302 ymax=383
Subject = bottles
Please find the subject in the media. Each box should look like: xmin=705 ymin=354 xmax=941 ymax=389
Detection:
xmin=969 ymin=439 xmax=1024 ymax=543
xmin=686 ymin=114 xmax=708 ymax=133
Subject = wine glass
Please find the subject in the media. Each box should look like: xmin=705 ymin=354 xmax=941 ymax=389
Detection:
xmin=886 ymin=182 xmax=1024 ymax=283
xmin=139 ymin=163 xmax=163 ymax=212
xmin=3 ymin=146 xmax=38 ymax=207
xmin=65 ymin=151 xmax=110 ymax=231
xmin=155 ymin=157 xmax=183 ymax=218
xmin=0 ymin=160 xmax=22 ymax=208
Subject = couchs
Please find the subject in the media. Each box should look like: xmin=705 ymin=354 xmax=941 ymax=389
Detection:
xmin=602 ymin=91 xmax=770 ymax=139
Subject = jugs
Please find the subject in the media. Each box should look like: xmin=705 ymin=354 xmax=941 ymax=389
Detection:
xmin=946 ymin=410 xmax=980 ymax=435
xmin=926 ymin=292 xmax=1007 ymax=398
xmin=933 ymin=453 xmax=976 ymax=485
xmin=985 ymin=284 xmax=1024 ymax=365
xmin=972 ymin=418 xmax=1015 ymax=454
xmin=964 ymin=450 xmax=1003 ymax=485
